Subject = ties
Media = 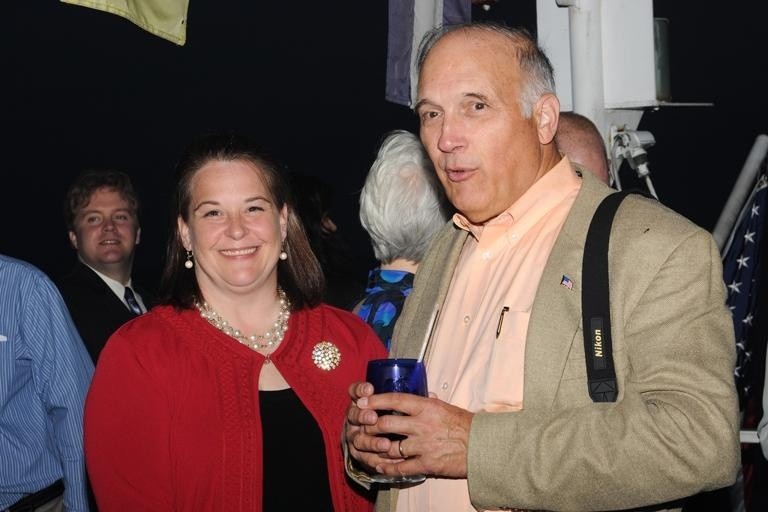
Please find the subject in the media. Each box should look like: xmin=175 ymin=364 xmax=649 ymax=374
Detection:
xmin=125 ymin=287 xmax=142 ymax=316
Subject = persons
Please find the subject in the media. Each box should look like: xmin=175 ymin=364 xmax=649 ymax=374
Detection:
xmin=555 ymin=111 xmax=612 ymax=188
xmin=342 ymin=25 xmax=743 ymax=511
xmin=354 ymin=130 xmax=458 ymax=351
xmin=83 ymin=147 xmax=388 ymax=512
xmin=52 ymin=164 xmax=164 ymax=366
xmin=1 ymin=255 xmax=96 ymax=509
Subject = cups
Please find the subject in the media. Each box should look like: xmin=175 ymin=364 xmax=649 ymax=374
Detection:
xmin=366 ymin=358 xmax=430 ymax=487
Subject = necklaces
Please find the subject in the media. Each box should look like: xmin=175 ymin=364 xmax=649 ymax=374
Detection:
xmin=192 ymin=282 xmax=290 ymax=349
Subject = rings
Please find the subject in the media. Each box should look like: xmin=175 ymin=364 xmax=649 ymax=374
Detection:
xmin=399 ymin=440 xmax=405 ymax=457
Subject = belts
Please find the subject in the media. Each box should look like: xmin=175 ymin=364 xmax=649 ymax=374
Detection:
xmin=8 ymin=477 xmax=64 ymax=512
